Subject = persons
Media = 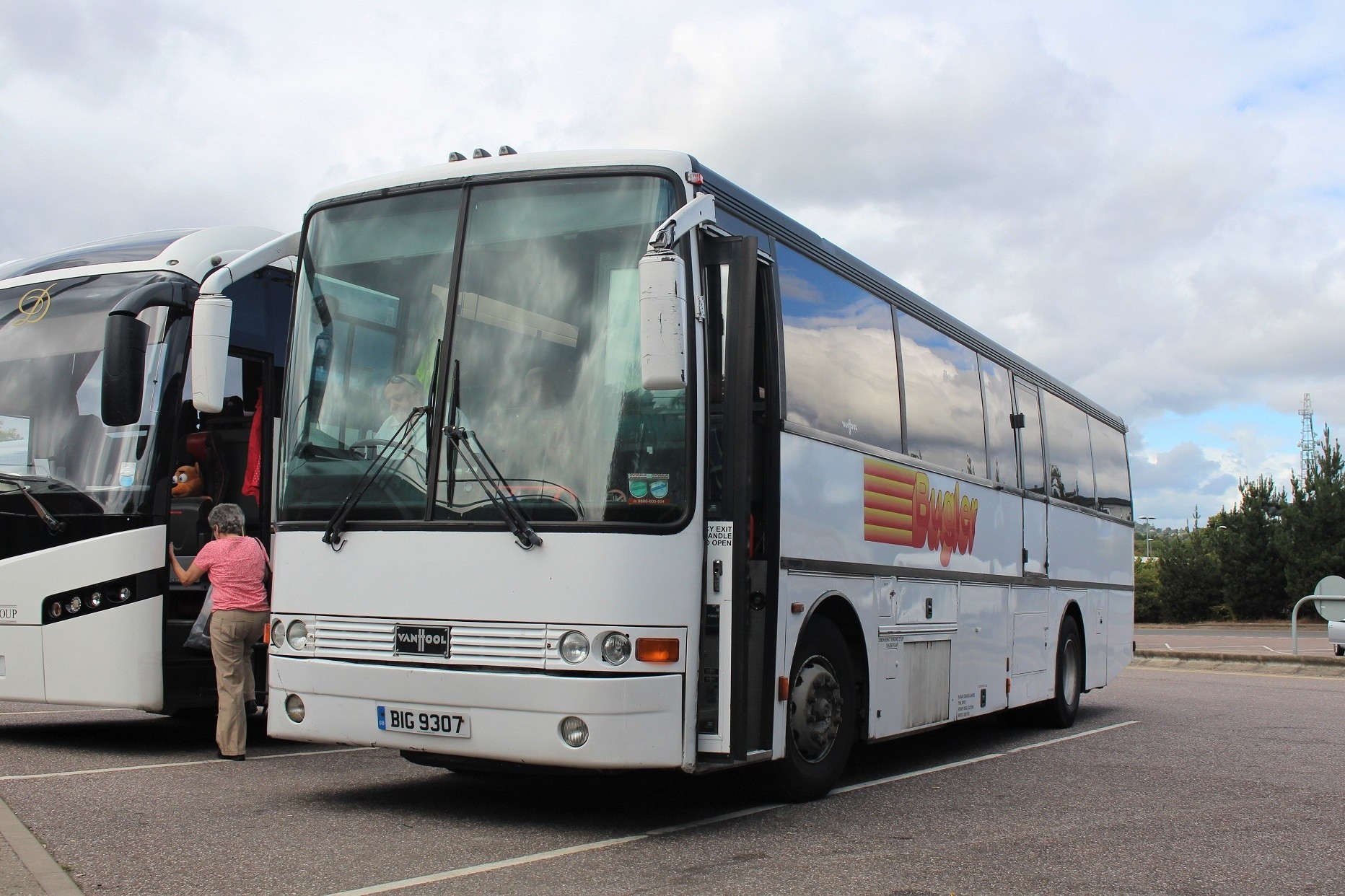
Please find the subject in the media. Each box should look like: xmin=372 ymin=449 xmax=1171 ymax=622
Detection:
xmin=169 ymin=502 xmax=272 ymax=761
xmin=363 ymin=374 xmax=483 ymax=460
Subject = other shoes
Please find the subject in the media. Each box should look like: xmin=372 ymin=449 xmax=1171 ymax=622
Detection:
xmin=217 ymin=749 xmax=246 ymax=761
xmin=244 ymin=699 xmax=258 ymax=716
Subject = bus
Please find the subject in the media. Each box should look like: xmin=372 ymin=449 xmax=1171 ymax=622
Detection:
xmin=0 ymin=223 xmax=580 ymax=725
xmin=189 ymin=144 xmax=1137 ymax=804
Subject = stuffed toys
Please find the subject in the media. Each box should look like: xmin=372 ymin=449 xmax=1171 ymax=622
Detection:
xmin=171 ymin=462 xmax=204 ymax=498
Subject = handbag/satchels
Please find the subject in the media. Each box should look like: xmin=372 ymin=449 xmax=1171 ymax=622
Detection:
xmin=253 ymin=537 xmax=273 ymax=609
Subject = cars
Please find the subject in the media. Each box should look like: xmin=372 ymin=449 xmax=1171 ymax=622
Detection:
xmin=1328 ymin=619 xmax=1345 ymax=656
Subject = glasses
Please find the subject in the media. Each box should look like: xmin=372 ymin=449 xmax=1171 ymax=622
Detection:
xmin=385 ymin=375 xmax=417 ymax=389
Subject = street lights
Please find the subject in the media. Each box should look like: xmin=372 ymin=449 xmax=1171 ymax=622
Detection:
xmin=1148 ymin=538 xmax=1155 ymax=562
xmin=1217 ymin=525 xmax=1227 ymax=549
xmin=1139 ymin=516 xmax=1156 ymax=559
